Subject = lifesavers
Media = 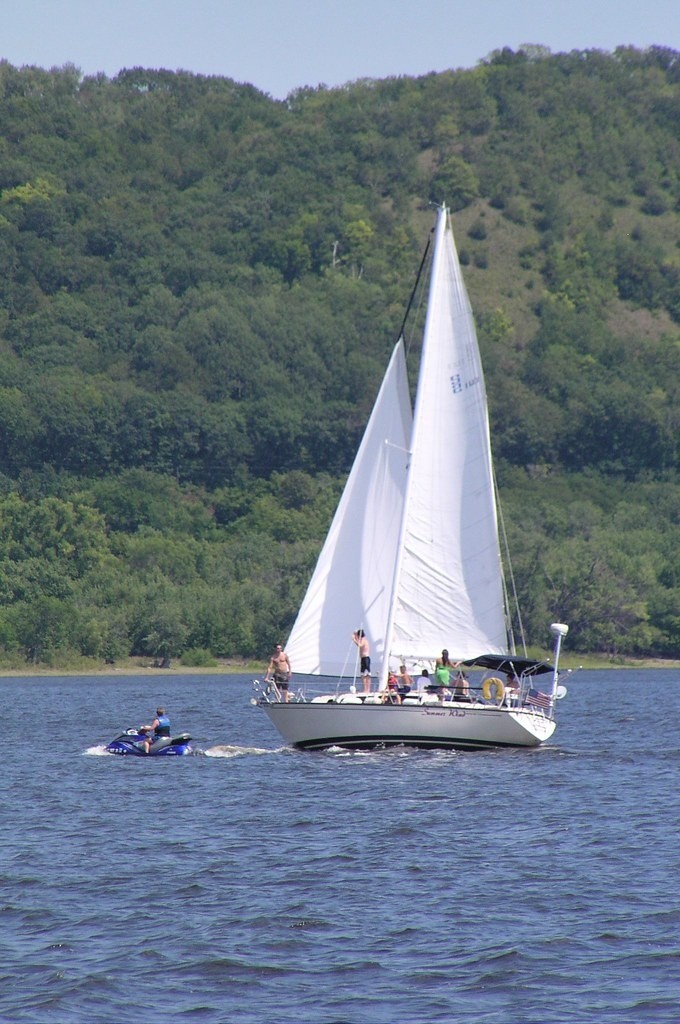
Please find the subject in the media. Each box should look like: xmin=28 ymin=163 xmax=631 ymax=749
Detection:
xmin=482 ymin=677 xmax=505 ymax=700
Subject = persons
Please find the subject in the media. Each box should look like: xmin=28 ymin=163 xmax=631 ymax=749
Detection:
xmin=352 ymin=629 xmax=371 ymax=694
xmin=265 ymin=643 xmax=291 ymax=703
xmin=140 ymin=706 xmax=170 ymax=754
xmin=387 ymin=648 xmax=520 ymax=708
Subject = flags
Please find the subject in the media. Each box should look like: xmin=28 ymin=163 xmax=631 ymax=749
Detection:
xmin=525 ymin=688 xmax=551 ymax=710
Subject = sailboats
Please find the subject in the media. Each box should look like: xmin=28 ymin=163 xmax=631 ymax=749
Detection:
xmin=252 ymin=198 xmax=571 ymax=755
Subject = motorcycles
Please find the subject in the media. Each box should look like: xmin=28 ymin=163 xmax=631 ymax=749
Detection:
xmin=106 ymin=728 xmax=192 ymax=756
